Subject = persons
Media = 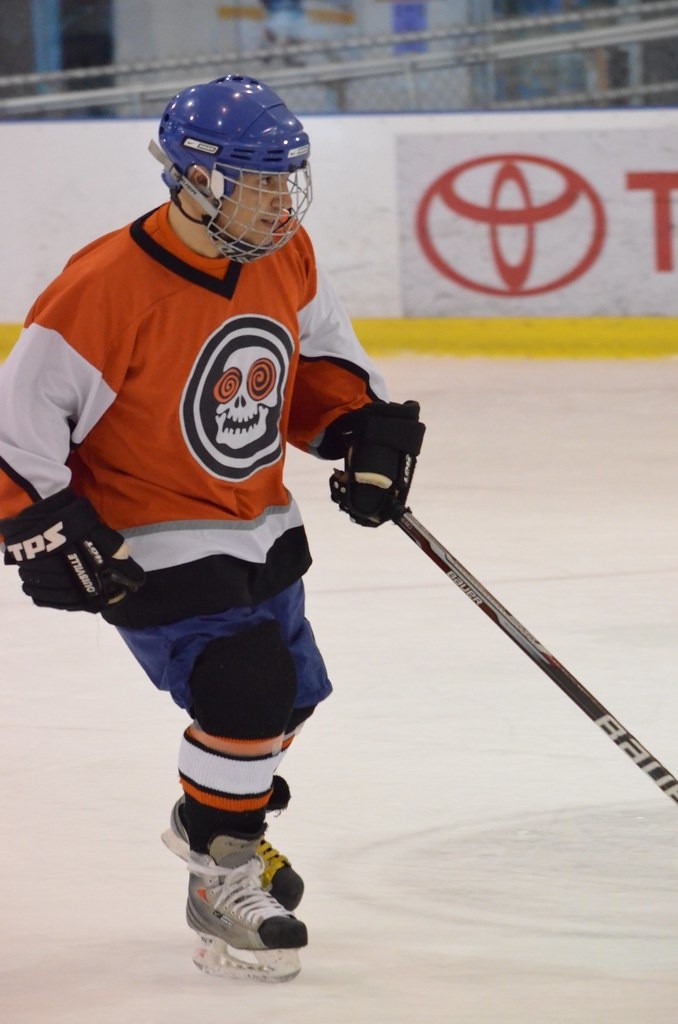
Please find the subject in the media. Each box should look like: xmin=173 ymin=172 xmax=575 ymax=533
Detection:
xmin=0 ymin=74 xmax=436 ymax=948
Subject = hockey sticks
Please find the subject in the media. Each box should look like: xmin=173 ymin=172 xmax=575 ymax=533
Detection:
xmin=397 ymin=511 xmax=678 ymax=801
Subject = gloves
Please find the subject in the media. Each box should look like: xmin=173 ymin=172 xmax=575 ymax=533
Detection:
xmin=2 ymin=494 xmax=147 ymax=616
xmin=332 ymin=396 xmax=427 ymax=525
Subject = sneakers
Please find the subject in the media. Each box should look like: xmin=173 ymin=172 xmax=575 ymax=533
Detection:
xmin=162 ymin=796 xmax=305 ymax=915
xmin=186 ymin=824 xmax=305 ymax=954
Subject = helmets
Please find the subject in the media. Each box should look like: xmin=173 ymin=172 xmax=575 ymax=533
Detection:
xmin=160 ymin=74 xmax=315 ymax=259
